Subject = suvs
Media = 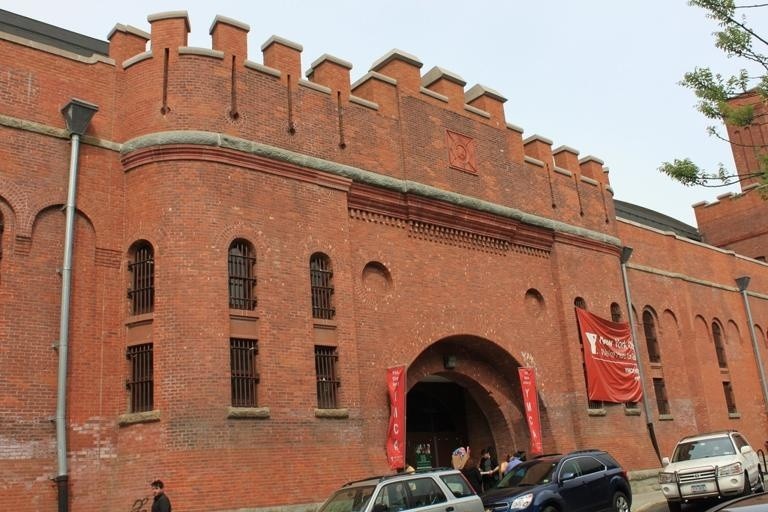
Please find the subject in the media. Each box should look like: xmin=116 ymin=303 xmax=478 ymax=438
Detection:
xmin=480 ymin=446 xmax=632 ymax=512
xmin=315 ymin=465 xmax=486 ymax=512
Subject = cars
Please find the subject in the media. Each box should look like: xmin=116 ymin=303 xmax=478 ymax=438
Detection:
xmin=657 ymin=429 xmax=768 ymax=512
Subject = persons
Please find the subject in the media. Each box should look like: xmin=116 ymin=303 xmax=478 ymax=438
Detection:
xmin=150 ymin=480 xmax=171 ymax=512
xmin=405 ymin=461 xmax=416 ymax=494
xmin=452 ymin=446 xmax=522 ymax=494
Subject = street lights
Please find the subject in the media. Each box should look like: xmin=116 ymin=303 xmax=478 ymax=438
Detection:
xmin=618 ymin=245 xmax=664 ymax=469
xmin=56 ymin=97 xmax=99 ymax=512
xmin=734 ymin=275 xmax=768 ymax=404
